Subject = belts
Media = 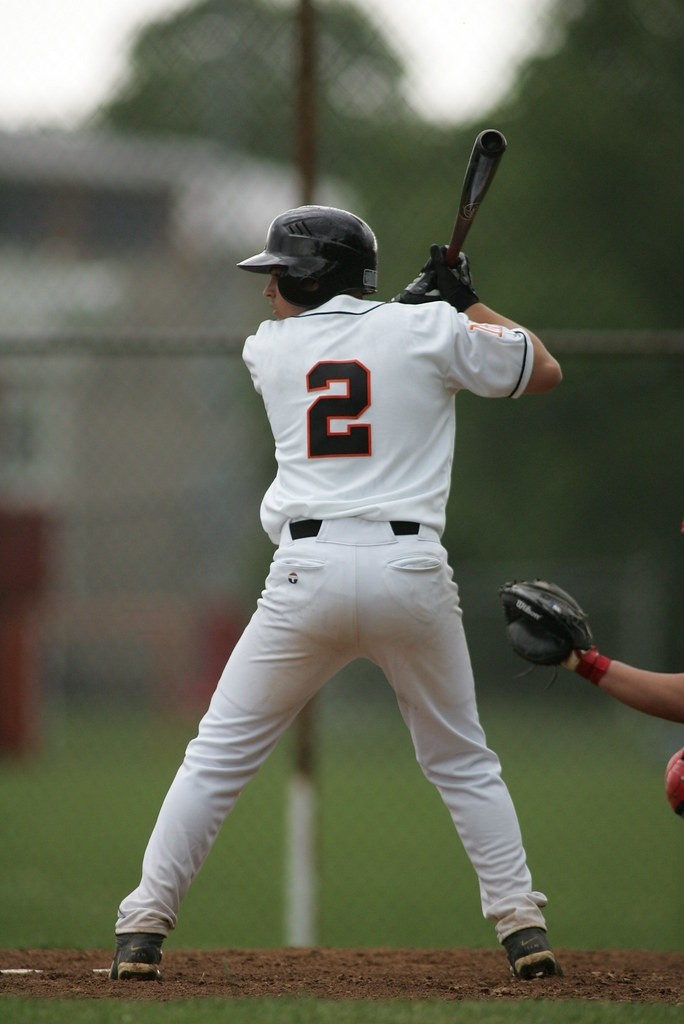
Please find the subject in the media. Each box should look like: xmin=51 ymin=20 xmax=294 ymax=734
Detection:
xmin=289 ymin=519 xmax=419 ymax=541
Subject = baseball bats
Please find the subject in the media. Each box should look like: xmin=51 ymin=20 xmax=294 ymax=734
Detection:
xmin=446 ymin=129 xmax=507 ymax=267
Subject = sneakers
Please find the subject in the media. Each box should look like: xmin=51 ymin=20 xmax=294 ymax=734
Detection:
xmin=504 ymin=931 xmax=561 ymax=980
xmin=109 ymin=930 xmax=162 ymax=982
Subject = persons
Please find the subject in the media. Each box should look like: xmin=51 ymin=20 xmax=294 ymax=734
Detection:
xmin=498 ymin=579 xmax=683 ymax=815
xmin=109 ymin=205 xmax=573 ymax=983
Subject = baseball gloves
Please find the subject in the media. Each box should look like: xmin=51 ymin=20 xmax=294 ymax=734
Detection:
xmin=498 ymin=578 xmax=593 ymax=668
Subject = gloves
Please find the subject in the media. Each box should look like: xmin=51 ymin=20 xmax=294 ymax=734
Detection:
xmin=388 ymin=243 xmax=480 ymax=311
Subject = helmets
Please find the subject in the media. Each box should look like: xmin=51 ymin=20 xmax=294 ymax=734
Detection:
xmin=237 ymin=207 xmax=377 ymax=308
